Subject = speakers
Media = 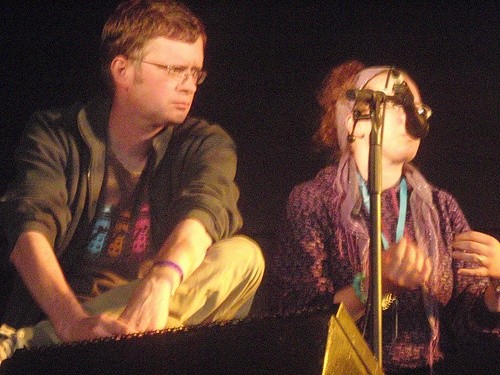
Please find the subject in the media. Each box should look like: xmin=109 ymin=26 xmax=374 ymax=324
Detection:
xmin=8 ymin=301 xmax=387 ymax=375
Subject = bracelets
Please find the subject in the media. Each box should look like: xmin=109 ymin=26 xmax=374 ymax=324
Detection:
xmin=354 ymin=270 xmax=394 ymax=310
xmin=149 ymin=259 xmax=184 ymax=286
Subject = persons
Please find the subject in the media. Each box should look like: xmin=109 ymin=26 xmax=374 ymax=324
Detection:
xmin=0 ymin=0 xmax=264 ymax=366
xmin=265 ymin=60 xmax=500 ymax=375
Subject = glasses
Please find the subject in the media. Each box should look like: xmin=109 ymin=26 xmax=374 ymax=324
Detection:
xmin=379 ymin=103 xmax=432 ymax=120
xmin=126 ymin=56 xmax=207 ymax=84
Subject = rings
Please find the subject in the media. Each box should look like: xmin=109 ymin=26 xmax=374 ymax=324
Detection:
xmin=478 ymin=255 xmax=483 ymax=264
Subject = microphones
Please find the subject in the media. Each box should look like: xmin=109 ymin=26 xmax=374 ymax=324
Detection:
xmin=392 ymin=69 xmax=429 ymax=138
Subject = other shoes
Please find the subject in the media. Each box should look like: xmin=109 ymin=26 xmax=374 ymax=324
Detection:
xmin=0 ymin=324 xmax=35 ymax=366
